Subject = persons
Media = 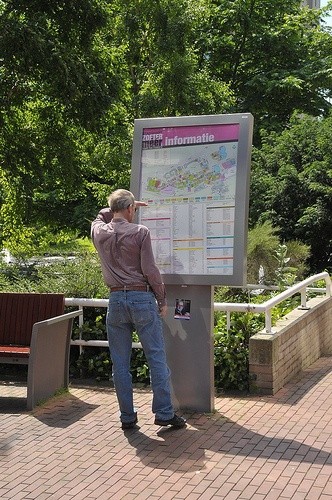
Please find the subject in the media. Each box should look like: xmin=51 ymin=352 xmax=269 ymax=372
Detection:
xmin=90 ymin=189 xmax=185 ymax=429
xmin=174 ymin=301 xmax=190 ymax=319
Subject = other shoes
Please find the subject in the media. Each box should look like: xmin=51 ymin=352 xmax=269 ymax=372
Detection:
xmin=122 ymin=422 xmax=138 ymax=431
xmin=154 ymin=415 xmax=185 ymax=426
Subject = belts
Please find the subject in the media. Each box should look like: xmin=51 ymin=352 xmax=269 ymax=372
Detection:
xmin=110 ymin=286 xmax=153 ymax=292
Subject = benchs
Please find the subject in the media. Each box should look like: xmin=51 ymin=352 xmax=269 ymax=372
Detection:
xmin=0 ymin=293 xmax=83 ymax=410
